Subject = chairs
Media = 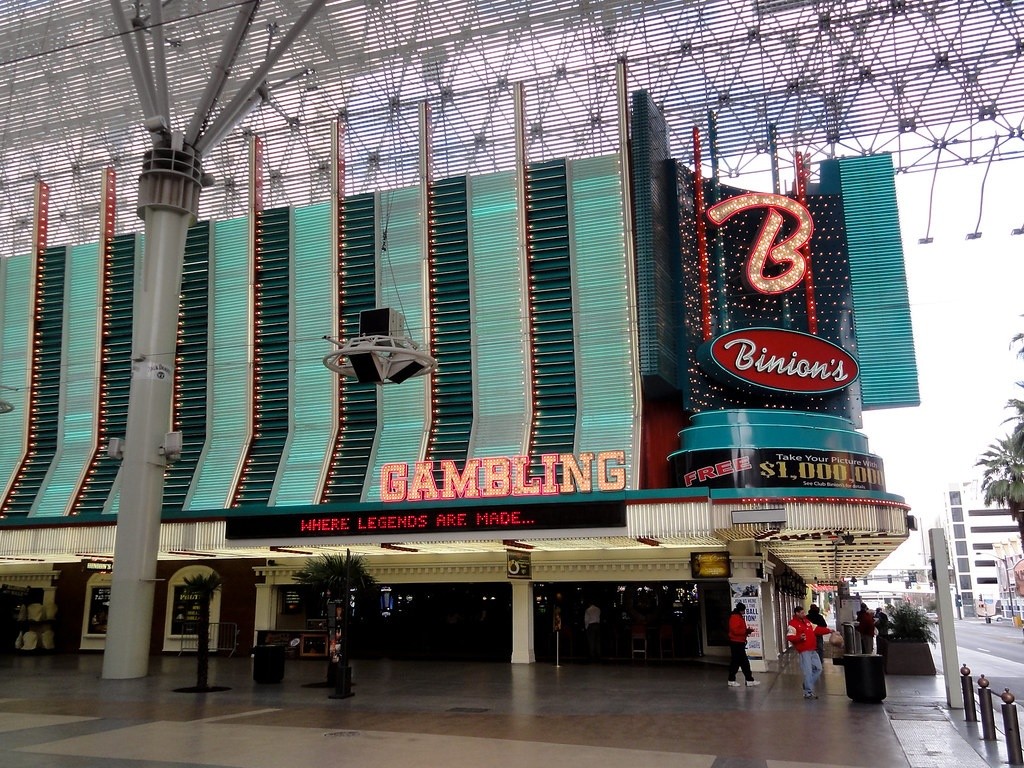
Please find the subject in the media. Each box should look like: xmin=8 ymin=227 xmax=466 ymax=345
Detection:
xmin=659 ymin=625 xmax=675 ymax=658
xmin=631 ymin=625 xmax=648 ymax=660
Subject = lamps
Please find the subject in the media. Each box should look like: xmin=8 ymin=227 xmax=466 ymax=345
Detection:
xmin=850 ymin=577 xmax=857 ymax=584
xmin=841 ymin=531 xmax=854 ymax=545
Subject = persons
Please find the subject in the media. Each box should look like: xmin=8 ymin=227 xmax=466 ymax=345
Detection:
xmin=786 ymin=606 xmax=835 ymax=699
xmin=856 ymin=603 xmax=876 ymax=655
xmin=874 ymin=607 xmax=890 ymax=634
xmin=583 ymin=598 xmax=601 ymax=660
xmin=727 ymin=602 xmax=760 ymax=686
xmin=805 ymin=603 xmax=827 ymax=668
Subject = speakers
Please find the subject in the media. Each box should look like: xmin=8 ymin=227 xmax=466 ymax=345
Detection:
xmin=348 ymin=308 xmax=425 ymax=384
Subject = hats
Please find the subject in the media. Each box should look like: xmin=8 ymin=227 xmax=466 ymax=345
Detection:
xmin=736 ymin=602 xmax=746 ymax=610
xmin=808 ymin=604 xmax=820 ymax=613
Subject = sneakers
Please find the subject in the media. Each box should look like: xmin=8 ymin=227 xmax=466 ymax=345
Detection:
xmin=728 ymin=681 xmax=740 ymax=686
xmin=803 ymin=690 xmax=818 ymax=699
xmin=746 ymin=681 xmax=760 ymax=686
